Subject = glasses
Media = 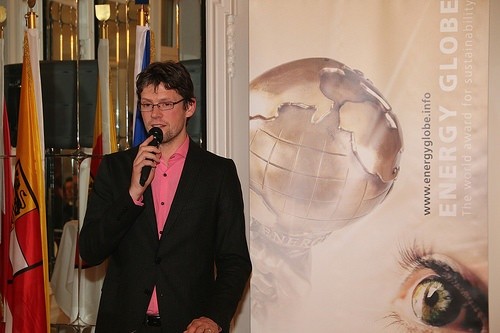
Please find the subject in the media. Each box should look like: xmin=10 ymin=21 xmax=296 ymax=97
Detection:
xmin=138 ymin=98 xmax=186 ymax=111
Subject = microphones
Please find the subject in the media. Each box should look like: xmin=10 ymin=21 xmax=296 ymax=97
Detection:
xmin=140 ymin=127 xmax=163 ymax=187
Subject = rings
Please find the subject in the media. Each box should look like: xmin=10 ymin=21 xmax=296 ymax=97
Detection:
xmin=205 ymin=328 xmax=211 ymax=333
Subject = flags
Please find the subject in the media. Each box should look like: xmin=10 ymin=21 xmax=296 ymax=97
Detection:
xmin=0 ymin=39 xmax=15 ymax=333
xmin=74 ymin=39 xmax=120 ymax=270
xmin=0 ymin=26 xmax=52 ymax=333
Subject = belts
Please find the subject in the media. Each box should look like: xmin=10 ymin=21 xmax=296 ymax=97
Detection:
xmin=144 ymin=315 xmax=161 ymax=327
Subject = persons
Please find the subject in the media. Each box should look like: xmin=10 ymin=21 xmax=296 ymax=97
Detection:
xmin=79 ymin=60 xmax=253 ymax=333
xmin=133 ymin=22 xmax=151 ymax=147
xmin=54 ymin=176 xmax=79 ymax=229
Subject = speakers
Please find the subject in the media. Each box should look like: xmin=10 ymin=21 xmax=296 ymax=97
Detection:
xmin=3 ymin=60 xmax=98 ymax=148
